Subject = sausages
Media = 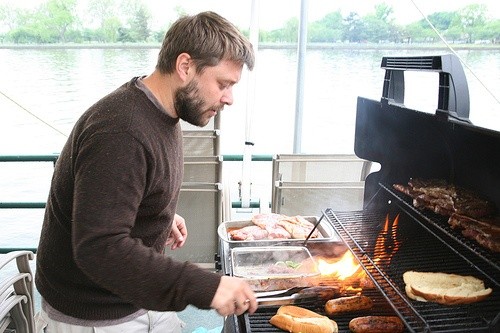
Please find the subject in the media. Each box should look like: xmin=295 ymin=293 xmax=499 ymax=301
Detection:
xmin=325 ymin=296 xmax=404 ymax=333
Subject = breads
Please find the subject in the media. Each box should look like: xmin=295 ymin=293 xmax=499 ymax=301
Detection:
xmin=402 ymin=270 xmax=492 ymax=307
xmin=268 ymin=305 xmax=338 ymax=333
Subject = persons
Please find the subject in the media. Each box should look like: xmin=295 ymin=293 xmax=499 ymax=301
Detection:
xmin=32 ymin=9 xmax=259 ymax=333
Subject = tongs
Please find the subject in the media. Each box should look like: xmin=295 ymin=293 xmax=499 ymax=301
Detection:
xmin=234 ymin=285 xmax=338 ymax=309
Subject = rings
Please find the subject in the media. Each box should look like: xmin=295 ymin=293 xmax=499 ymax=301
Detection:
xmin=243 ymin=299 xmax=250 ymax=302
